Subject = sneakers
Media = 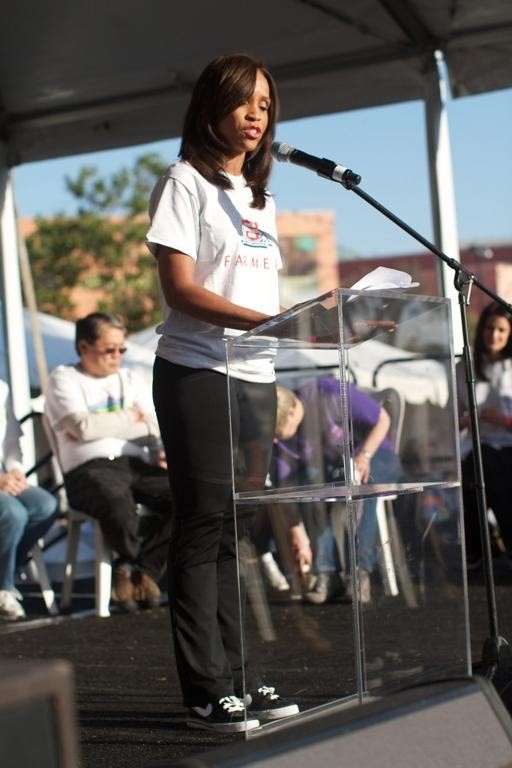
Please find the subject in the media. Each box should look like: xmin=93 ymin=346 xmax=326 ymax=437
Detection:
xmin=262 ymin=555 xmax=293 ymax=595
xmin=186 ymin=693 xmax=259 ymax=734
xmin=303 ymin=573 xmax=331 ymax=604
xmin=1 ymin=586 xmax=27 ymax=621
xmin=346 ymin=567 xmax=372 ymax=603
xmin=237 ymin=685 xmax=301 ymax=722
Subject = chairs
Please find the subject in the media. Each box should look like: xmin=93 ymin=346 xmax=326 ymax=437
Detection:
xmin=40 ymin=409 xmax=162 ymax=619
xmin=291 ymin=387 xmax=404 ymax=602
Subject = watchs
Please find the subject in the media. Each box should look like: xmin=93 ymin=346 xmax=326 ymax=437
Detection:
xmin=357 ymin=449 xmax=372 ymax=460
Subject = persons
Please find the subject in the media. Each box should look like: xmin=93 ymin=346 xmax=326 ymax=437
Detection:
xmin=148 ymin=53 xmax=307 ymax=738
xmin=0 ymin=376 xmax=60 ymax=628
xmin=267 ymin=364 xmax=403 ymax=612
xmin=37 ymin=308 xmax=175 ymax=618
xmin=440 ymin=299 xmax=510 ymax=587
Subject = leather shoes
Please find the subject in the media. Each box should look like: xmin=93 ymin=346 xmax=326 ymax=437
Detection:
xmin=137 ymin=567 xmax=162 ymax=607
xmin=112 ymin=568 xmax=138 ymax=614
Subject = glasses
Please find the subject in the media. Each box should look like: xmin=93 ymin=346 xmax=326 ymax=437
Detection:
xmin=85 ymin=341 xmax=128 ymax=356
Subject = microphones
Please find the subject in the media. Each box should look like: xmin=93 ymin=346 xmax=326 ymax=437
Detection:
xmin=271 ymin=141 xmax=362 ymax=185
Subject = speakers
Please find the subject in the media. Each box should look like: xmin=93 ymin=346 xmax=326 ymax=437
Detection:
xmin=187 ymin=678 xmax=512 ymax=768
